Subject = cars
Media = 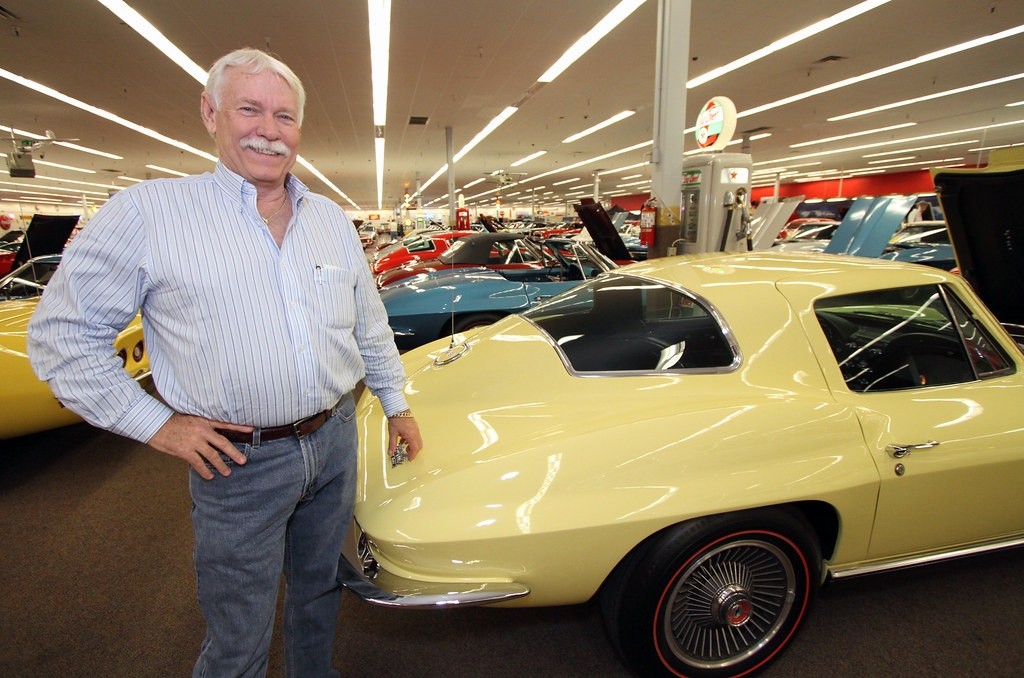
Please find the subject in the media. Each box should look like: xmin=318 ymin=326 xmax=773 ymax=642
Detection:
xmin=339 ymin=145 xmax=1024 ymax=662
xmin=0 ymin=220 xmax=960 ymax=441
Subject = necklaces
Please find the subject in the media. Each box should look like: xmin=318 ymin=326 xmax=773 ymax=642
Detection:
xmin=261 ymin=189 xmax=287 ymax=224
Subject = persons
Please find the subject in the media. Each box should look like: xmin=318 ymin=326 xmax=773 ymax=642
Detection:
xmin=907 ymin=200 xmax=928 ymax=223
xmin=26 ymin=47 xmax=424 ymax=678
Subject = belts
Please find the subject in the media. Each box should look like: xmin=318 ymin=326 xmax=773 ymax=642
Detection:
xmin=214 ymin=397 xmax=340 ymax=443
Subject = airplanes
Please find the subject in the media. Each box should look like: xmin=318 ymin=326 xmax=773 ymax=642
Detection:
xmin=1 ymin=130 xmax=81 ymax=158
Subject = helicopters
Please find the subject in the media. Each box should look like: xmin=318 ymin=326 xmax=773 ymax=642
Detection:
xmin=485 ymin=170 xmax=528 ymax=189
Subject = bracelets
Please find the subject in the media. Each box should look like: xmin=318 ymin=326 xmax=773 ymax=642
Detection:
xmin=393 ymin=413 xmax=415 ymax=417
